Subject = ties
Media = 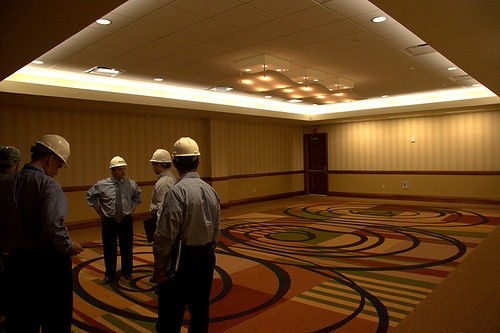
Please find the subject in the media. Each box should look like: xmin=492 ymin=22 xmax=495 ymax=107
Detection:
xmin=116 ymin=181 xmax=122 ymax=224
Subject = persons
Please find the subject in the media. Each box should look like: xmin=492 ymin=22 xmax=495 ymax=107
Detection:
xmin=0 ymin=144 xmax=22 ymax=333
xmin=87 ymin=156 xmax=142 ymax=284
xmin=153 ymin=136 xmax=220 ymax=333
xmin=0 ymin=135 xmax=84 ymax=333
xmin=149 ymin=149 xmax=177 ymax=292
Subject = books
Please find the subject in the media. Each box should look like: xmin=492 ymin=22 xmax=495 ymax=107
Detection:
xmin=151 ymin=238 xmax=182 ymax=283
xmin=144 ymin=218 xmax=157 ymax=243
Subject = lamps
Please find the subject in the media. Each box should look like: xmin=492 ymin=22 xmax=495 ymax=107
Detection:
xmin=215 ymin=64 xmax=368 ymax=105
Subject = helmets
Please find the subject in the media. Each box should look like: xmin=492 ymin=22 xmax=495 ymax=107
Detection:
xmin=149 ymin=149 xmax=172 ymax=163
xmin=108 ymin=155 xmax=128 ymax=168
xmin=174 ymin=137 xmax=201 ymax=156
xmin=36 ymin=134 xmax=70 ymax=168
xmin=0 ymin=146 xmax=21 ymax=164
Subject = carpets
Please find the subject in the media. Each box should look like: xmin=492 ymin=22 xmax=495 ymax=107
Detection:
xmin=70 ymin=200 xmax=500 ymax=333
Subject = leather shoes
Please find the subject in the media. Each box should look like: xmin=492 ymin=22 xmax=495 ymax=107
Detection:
xmin=102 ymin=275 xmax=114 ymax=283
xmin=124 ymin=273 xmax=132 ymax=281
